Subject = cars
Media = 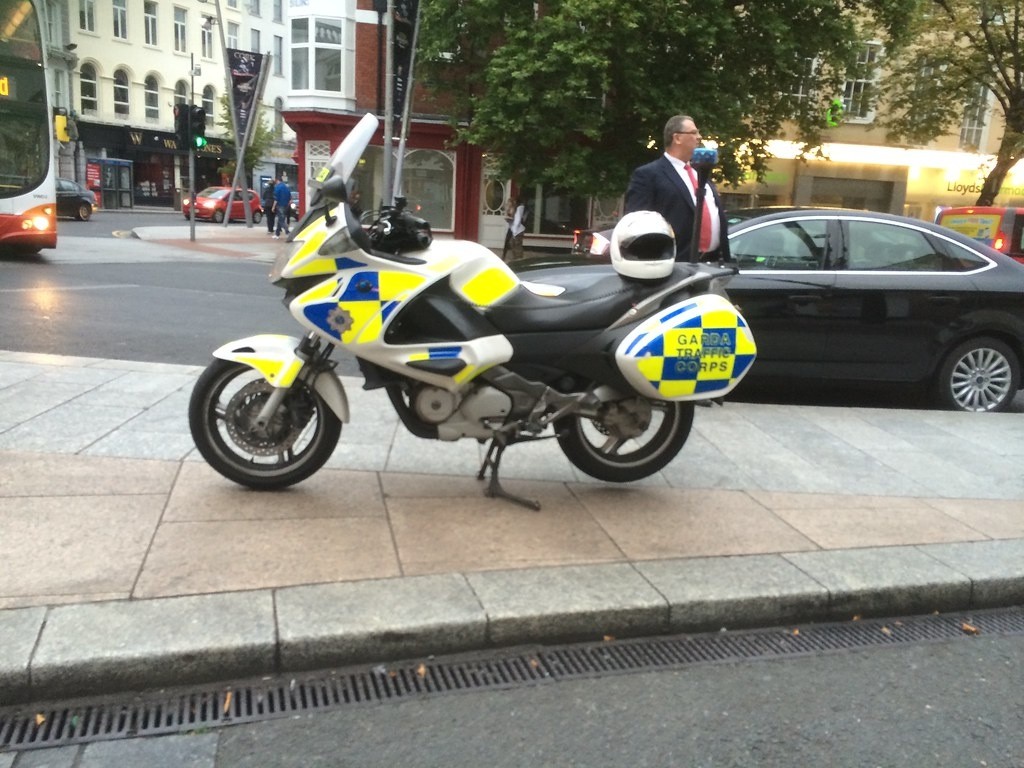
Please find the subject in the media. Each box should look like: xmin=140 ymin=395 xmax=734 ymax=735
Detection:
xmin=287 ymin=192 xmax=300 ymax=221
xmin=571 ymin=222 xmax=618 ymax=264
xmin=182 ymin=185 xmax=263 ymax=228
xmin=55 ymin=178 xmax=98 ymax=221
xmin=722 ymin=206 xmax=1024 ymax=413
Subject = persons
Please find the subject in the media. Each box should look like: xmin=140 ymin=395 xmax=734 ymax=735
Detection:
xmin=624 ymin=115 xmax=732 ymax=268
xmin=501 ymin=196 xmax=527 ymax=261
xmin=349 ymin=184 xmax=362 ymax=217
xmin=263 ymin=178 xmax=292 ymax=238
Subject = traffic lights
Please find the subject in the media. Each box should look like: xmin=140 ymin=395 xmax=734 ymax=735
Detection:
xmin=191 ymin=107 xmax=208 ymax=149
xmin=174 ymin=103 xmax=188 ymax=150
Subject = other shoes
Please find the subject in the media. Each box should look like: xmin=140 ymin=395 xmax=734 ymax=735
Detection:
xmin=272 ymin=235 xmax=280 ymax=239
xmin=286 ymin=233 xmax=290 ymax=239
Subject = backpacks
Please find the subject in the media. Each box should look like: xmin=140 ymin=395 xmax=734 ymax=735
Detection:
xmin=520 ymin=208 xmax=533 ymax=228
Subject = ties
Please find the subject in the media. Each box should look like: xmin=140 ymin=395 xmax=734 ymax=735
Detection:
xmin=684 ymin=164 xmax=711 ymax=253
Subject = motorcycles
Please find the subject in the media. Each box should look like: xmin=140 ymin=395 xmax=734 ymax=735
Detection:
xmin=188 ymin=109 xmax=758 ymax=512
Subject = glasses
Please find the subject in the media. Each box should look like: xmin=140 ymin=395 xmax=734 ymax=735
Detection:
xmin=675 ymin=130 xmax=699 ymax=136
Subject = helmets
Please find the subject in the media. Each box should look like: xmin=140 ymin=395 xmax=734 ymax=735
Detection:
xmin=610 ymin=210 xmax=677 ymax=281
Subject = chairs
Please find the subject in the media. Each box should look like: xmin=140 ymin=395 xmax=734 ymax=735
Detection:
xmin=866 ymin=242 xmax=918 ymax=264
xmin=759 ymin=232 xmax=784 ymax=254
xmin=798 ymin=234 xmax=826 ymax=260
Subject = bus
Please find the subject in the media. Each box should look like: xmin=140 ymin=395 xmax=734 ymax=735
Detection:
xmin=933 ymin=206 xmax=1024 ymax=265
xmin=0 ymin=0 xmax=71 ymax=255
